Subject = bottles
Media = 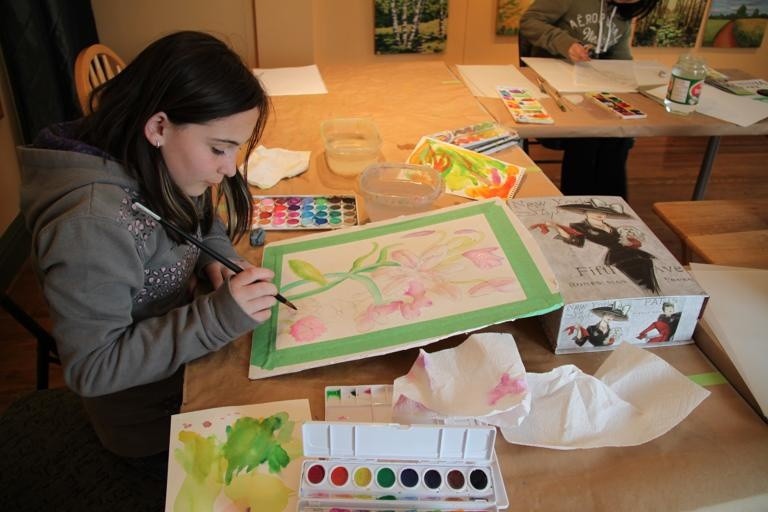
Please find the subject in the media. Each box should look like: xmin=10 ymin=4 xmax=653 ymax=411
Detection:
xmin=661 ymin=52 xmax=707 ymax=115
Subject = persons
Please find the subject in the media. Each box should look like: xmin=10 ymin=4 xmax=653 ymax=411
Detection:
xmin=517 ymin=0 xmax=661 ymax=204
xmin=15 ymin=29 xmax=278 ymax=479
xmin=541 ymin=199 xmax=661 ymax=299
xmin=569 ymin=305 xmax=628 ymax=346
xmin=636 ymin=301 xmax=683 ymax=347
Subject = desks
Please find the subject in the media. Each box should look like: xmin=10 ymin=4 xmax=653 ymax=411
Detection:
xmin=182 ymin=60 xmax=766 ymax=508
xmin=449 ymin=61 xmax=766 ymax=201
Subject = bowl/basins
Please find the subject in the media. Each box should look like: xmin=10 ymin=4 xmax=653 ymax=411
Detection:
xmin=356 ymin=163 xmax=443 ymax=223
xmin=318 ymin=117 xmax=380 ymax=177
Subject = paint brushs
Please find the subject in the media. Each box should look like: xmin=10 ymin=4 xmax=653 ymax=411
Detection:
xmin=537 ymin=77 xmax=567 ymax=112
xmin=134 ymin=202 xmax=298 ymax=310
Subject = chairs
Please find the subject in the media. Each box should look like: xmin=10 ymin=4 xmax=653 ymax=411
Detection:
xmin=73 ymin=43 xmax=127 ymax=118
xmin=0 ymin=210 xmax=167 ymax=512
xmin=518 ymin=30 xmax=564 ymax=164
